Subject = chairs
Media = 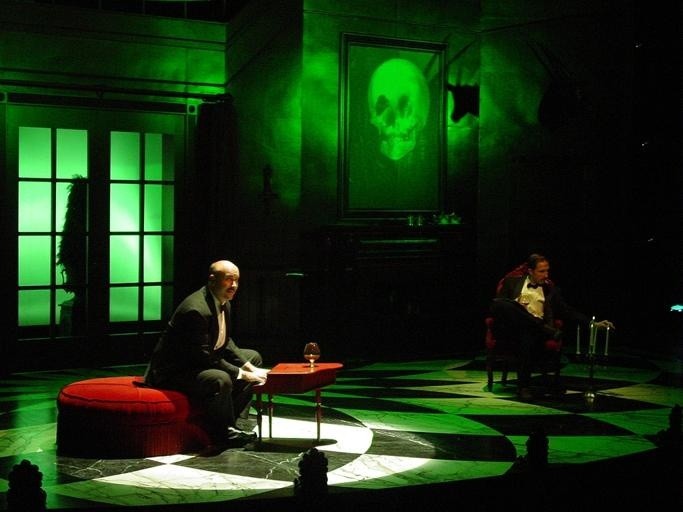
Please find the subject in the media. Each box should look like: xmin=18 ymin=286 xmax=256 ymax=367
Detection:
xmin=484 ymin=260 xmax=561 ymax=392
xmin=56 ymin=375 xmax=191 ymax=460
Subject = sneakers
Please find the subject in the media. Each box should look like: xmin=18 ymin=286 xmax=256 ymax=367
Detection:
xmin=517 ymin=386 xmax=533 ymax=401
xmin=549 ymin=327 xmax=564 ymax=342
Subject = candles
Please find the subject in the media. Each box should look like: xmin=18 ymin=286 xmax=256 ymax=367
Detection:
xmin=575 ymin=315 xmax=610 ymax=357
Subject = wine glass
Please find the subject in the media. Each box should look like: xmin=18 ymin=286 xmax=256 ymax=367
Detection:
xmin=518 ymin=292 xmax=530 ymax=311
xmin=304 ymin=342 xmax=321 ymax=368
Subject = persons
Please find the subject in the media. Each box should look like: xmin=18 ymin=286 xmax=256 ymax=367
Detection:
xmin=140 ymin=257 xmax=271 ymax=458
xmin=491 ymin=252 xmax=615 ymax=400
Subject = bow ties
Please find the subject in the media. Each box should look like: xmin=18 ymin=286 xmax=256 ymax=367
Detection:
xmin=527 ymin=283 xmax=538 ymax=289
xmin=219 ymin=302 xmax=227 ymax=314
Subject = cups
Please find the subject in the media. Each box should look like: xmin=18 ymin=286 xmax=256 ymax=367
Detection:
xmin=406 ymin=214 xmax=426 ymax=227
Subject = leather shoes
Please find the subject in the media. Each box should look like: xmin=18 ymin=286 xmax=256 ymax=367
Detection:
xmin=219 ymin=427 xmax=257 ymax=448
